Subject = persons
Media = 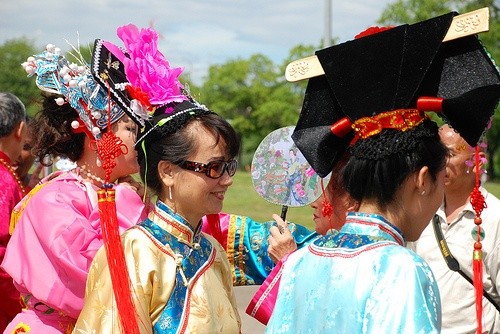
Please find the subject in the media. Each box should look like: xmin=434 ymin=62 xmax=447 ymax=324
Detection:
xmin=262 ymin=113 xmax=450 ymax=334
xmin=414 ymin=124 xmax=500 ymax=334
xmin=70 ymin=109 xmax=244 ymax=334
xmin=0 ymin=92 xmax=151 ymax=334
xmin=203 ymin=166 xmax=361 ymax=325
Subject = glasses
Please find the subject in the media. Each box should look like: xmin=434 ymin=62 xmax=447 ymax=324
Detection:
xmin=176 ymin=158 xmax=237 ymax=179
